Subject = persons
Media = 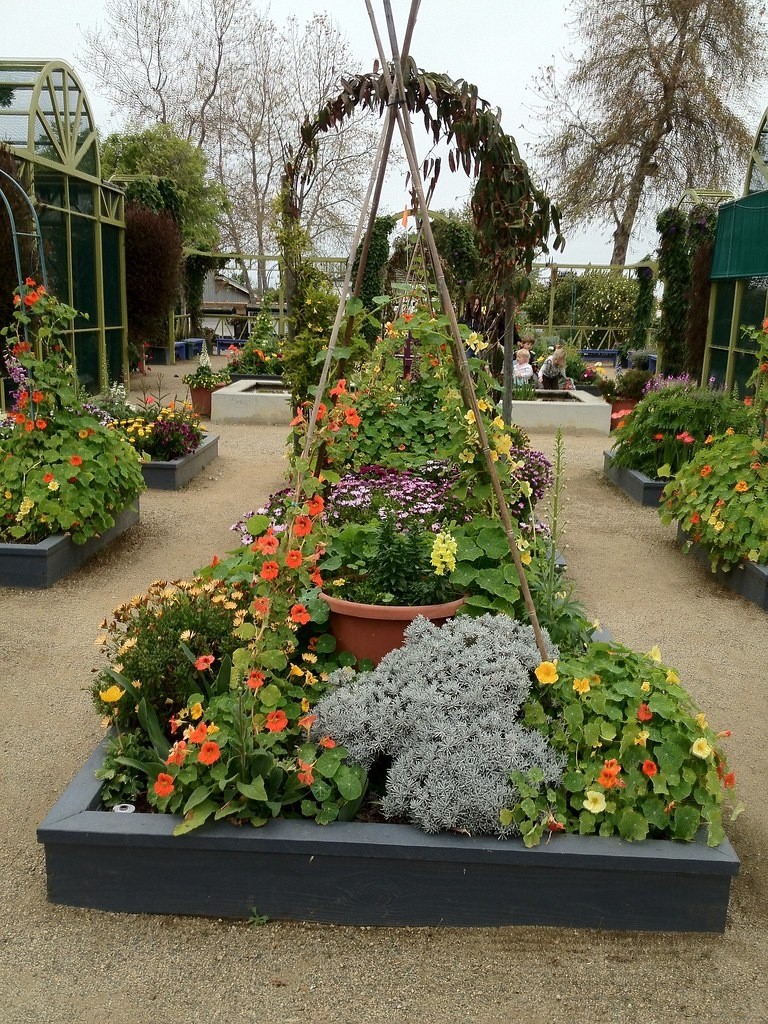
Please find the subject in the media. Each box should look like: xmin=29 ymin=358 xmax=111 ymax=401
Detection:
xmin=457 ymin=295 xmax=522 ymax=377
xmin=513 ymin=336 xmax=576 ymax=390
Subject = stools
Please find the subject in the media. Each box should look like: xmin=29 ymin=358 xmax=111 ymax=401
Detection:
xmin=174 ymin=342 xmax=186 ymax=361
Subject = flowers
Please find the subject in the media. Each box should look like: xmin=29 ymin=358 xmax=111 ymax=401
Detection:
xmin=0 ymin=266 xmax=768 ymax=849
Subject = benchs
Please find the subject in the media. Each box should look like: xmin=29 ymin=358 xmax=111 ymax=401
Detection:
xmin=183 ymin=338 xmax=205 ymax=360
xmin=216 ymin=339 xmax=248 ymax=356
xmin=579 ymin=349 xmax=617 ymax=367
xmin=649 ymin=355 xmax=657 ymax=374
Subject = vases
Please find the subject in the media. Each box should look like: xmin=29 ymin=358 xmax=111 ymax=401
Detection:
xmin=190 ymin=382 xmax=226 ymax=415
xmin=610 ymin=399 xmax=641 ymax=435
xmin=317 ymin=576 xmax=472 ymax=670
